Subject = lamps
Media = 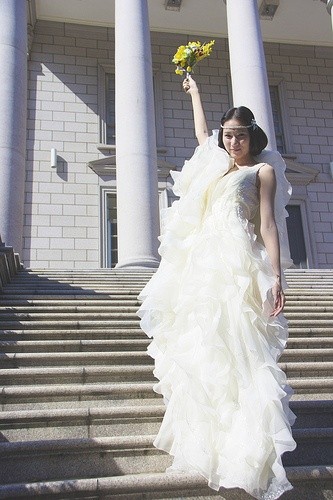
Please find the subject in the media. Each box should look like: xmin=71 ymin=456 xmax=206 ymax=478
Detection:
xmin=50 ymin=149 xmax=57 ymax=168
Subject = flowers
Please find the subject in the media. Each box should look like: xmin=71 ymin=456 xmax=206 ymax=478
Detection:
xmin=172 ymin=40 xmax=215 ymax=80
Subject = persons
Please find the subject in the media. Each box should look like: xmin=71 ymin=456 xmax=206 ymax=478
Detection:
xmin=136 ymin=75 xmax=297 ymax=500
